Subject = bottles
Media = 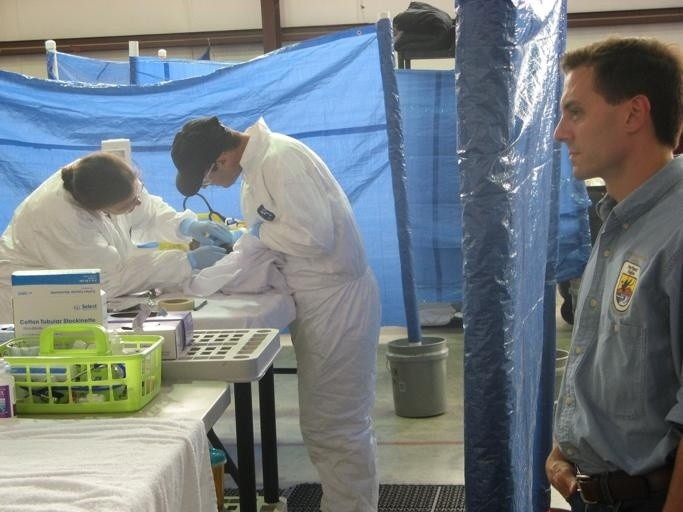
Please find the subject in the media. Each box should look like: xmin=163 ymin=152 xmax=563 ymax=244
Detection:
xmin=0 ymin=359 xmax=15 ymax=426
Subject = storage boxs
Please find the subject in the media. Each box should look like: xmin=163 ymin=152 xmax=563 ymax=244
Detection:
xmin=8 ymin=269 xmax=108 ymax=360
xmin=209 ymin=447 xmax=228 ymax=512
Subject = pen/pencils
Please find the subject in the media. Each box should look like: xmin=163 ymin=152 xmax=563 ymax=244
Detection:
xmin=194 ymin=300 xmax=208 ymax=311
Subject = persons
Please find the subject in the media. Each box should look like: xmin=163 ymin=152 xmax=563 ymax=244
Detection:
xmin=170 ymin=114 xmax=384 ymax=511
xmin=540 ymin=35 xmax=683 ymax=509
xmin=0 ymin=149 xmax=235 ymax=331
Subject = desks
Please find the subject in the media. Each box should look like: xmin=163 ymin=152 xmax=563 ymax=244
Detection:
xmin=0 ymin=381 xmax=231 ymax=512
xmin=105 ymin=284 xmax=298 ymax=512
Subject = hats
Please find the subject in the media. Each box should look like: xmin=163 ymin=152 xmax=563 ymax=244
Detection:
xmin=171 ymin=115 xmax=225 ymax=196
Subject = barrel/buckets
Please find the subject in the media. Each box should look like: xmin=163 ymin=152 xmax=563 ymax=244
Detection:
xmin=555 ymin=348 xmax=569 ymax=400
xmin=385 ymin=338 xmax=450 ymax=417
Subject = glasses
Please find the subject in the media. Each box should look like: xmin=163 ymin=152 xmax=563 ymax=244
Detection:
xmin=107 ymin=181 xmax=144 ymax=215
xmin=201 ymin=163 xmax=217 ymax=187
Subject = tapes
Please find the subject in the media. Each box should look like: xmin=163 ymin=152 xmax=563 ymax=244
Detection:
xmin=158 ymin=298 xmax=195 ymax=311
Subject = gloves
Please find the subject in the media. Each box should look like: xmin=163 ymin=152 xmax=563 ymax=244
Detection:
xmin=179 ymin=218 xmax=241 ymax=245
xmin=189 ymin=242 xmax=227 ymax=269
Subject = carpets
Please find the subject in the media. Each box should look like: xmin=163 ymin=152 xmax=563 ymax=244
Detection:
xmin=220 ymin=482 xmax=467 ymax=512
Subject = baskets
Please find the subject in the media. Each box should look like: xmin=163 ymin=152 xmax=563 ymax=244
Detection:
xmin=0 ymin=323 xmax=164 ymax=413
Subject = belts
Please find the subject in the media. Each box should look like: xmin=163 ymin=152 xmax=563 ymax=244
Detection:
xmin=576 ymin=468 xmax=674 ymax=504
